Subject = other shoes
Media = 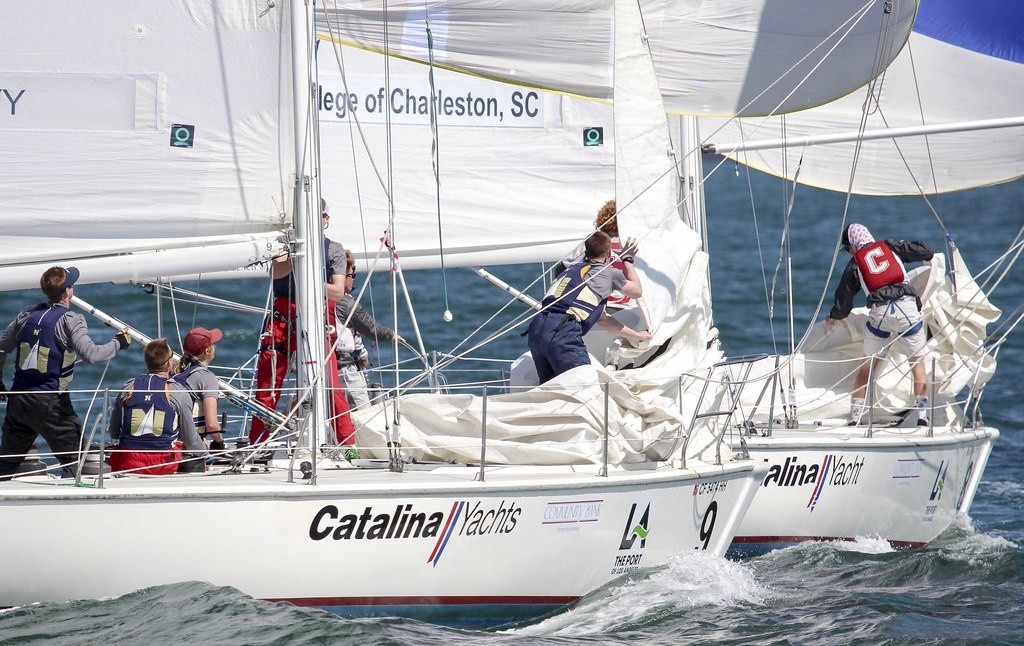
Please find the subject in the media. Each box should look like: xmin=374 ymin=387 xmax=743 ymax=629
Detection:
xmin=846 ymin=418 xmax=862 ymax=426
xmin=344 ymin=448 xmax=360 ymax=462
xmin=917 ymin=416 xmax=931 ymax=427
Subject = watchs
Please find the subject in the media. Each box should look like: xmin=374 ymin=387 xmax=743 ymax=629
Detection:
xmin=622 ymin=256 xmax=634 ymax=263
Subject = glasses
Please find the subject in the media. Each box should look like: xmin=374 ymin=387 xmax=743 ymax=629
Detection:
xmin=345 ymin=272 xmax=356 ymax=279
xmin=352 ymin=266 xmax=356 ymax=272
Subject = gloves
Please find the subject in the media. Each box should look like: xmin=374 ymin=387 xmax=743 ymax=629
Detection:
xmin=0 ymin=382 xmax=9 ymax=401
xmin=209 ymin=440 xmax=225 ymax=454
xmin=114 ymin=326 xmax=131 ymax=350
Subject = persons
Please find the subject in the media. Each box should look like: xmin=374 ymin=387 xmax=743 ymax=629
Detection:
xmin=167 ymin=328 xmax=224 ymax=456
xmin=827 ymin=223 xmax=934 ymax=426
xmin=556 ymin=200 xmax=636 ymax=307
xmin=0 ymin=266 xmax=131 ymax=481
xmin=248 ymin=197 xmax=405 ymax=460
xmin=106 ymin=336 xmax=211 ymax=475
xmin=528 ymin=232 xmax=642 ymax=385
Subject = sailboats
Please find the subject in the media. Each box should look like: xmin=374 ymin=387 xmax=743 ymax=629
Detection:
xmin=0 ymin=0 xmax=1024 ymax=614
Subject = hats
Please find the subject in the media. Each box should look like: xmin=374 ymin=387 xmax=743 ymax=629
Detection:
xmin=838 ymin=224 xmax=852 ymax=252
xmin=321 ymin=198 xmax=330 ymax=217
xmin=47 ymin=267 xmax=80 ymax=298
xmin=183 ymin=327 xmax=223 ymax=357
